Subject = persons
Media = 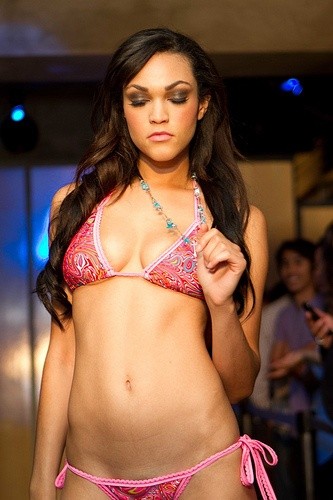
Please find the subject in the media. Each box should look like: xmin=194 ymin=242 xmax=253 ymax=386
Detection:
xmin=264 ymin=236 xmax=331 ymax=500
xmin=300 ymin=243 xmax=333 ymax=500
xmin=24 ymin=29 xmax=277 ymax=500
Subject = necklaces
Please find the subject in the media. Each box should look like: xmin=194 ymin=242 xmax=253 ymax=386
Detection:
xmin=133 ymin=163 xmax=208 ymax=260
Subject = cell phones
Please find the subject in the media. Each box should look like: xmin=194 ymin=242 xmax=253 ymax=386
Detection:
xmin=302 ymin=300 xmax=333 ymax=336
